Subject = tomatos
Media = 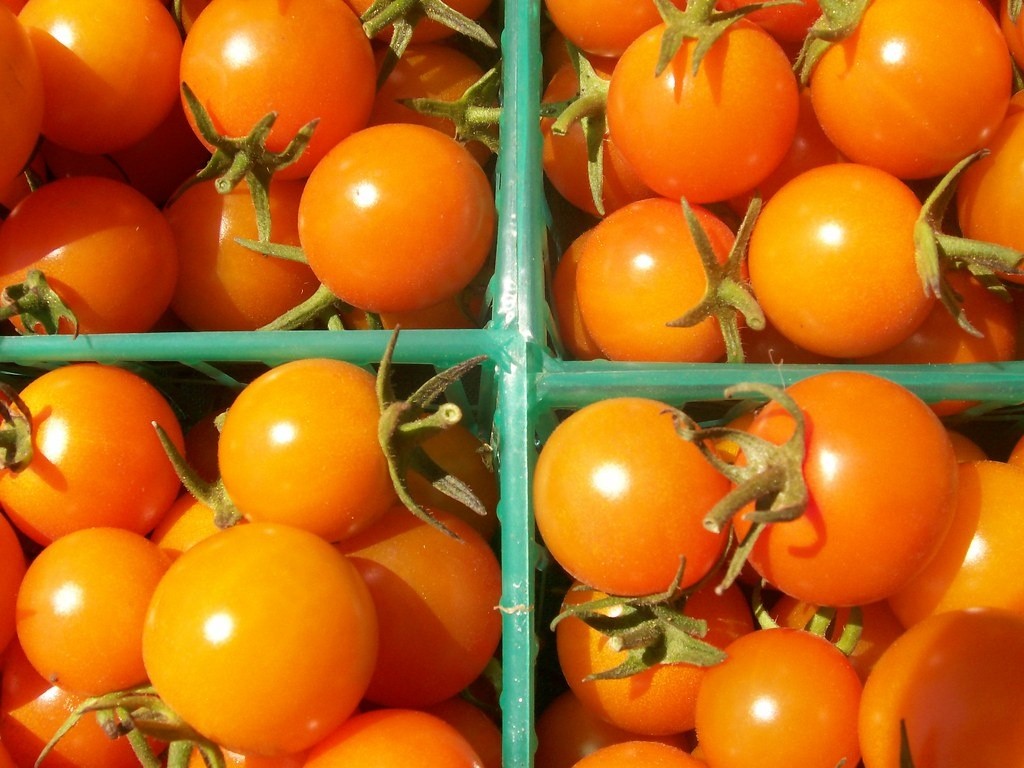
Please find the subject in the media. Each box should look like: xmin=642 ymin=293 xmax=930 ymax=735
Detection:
xmin=0 ymin=0 xmax=1024 ymax=768
